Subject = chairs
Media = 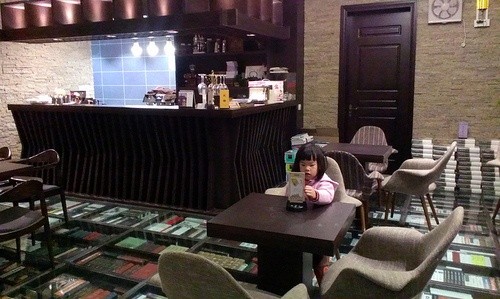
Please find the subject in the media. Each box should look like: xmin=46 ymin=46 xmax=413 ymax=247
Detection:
xmin=264 ymin=155 xmax=361 ymax=260
xmin=0 ymin=149 xmax=69 ymax=246
xmin=158 ymin=250 xmax=310 ymax=299
xmin=0 ymin=180 xmax=56 ymax=269
xmin=324 ymin=150 xmax=385 ymax=230
xmin=485 ymin=145 xmax=500 ymax=220
xmin=320 ymin=206 xmax=464 ymax=299
xmin=0 ymin=146 xmax=12 ymax=188
xmin=379 ymin=141 xmax=458 ymax=232
xmin=349 ymin=126 xmax=398 ymax=173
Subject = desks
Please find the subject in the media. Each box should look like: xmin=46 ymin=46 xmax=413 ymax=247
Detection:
xmin=315 ymin=143 xmax=392 ymax=175
xmin=206 ymin=193 xmax=355 ymax=299
xmin=1 ymin=161 xmax=33 ymax=185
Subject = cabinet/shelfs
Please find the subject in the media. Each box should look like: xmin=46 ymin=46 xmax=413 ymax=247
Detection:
xmin=174 ymin=32 xmax=270 ymax=103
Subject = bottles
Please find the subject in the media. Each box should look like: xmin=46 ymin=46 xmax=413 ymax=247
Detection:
xmin=267 ymin=85 xmax=274 ymax=101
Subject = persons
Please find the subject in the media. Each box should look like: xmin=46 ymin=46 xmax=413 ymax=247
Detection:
xmin=286 ymin=143 xmax=339 ymax=288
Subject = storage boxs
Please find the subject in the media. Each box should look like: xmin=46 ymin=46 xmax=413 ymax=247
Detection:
xmin=285 ymin=133 xmax=314 ymax=183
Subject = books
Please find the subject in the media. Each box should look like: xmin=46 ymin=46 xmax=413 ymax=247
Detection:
xmin=311 ymin=138 xmax=500 ymax=299
xmin=0 ymin=199 xmax=257 ymax=299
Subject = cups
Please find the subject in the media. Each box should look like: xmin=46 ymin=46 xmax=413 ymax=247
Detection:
xmin=458 ymin=120 xmax=469 ymax=138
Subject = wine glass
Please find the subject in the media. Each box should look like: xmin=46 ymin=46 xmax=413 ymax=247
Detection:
xmin=208 ymin=75 xmax=228 ymax=96
xmin=198 ymin=74 xmax=208 ymax=95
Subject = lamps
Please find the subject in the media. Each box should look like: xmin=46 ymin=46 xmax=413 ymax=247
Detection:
xmin=147 ymin=38 xmax=159 ymax=57
xmin=164 ymin=37 xmax=175 ymax=56
xmin=131 ymin=39 xmax=143 ymax=57
